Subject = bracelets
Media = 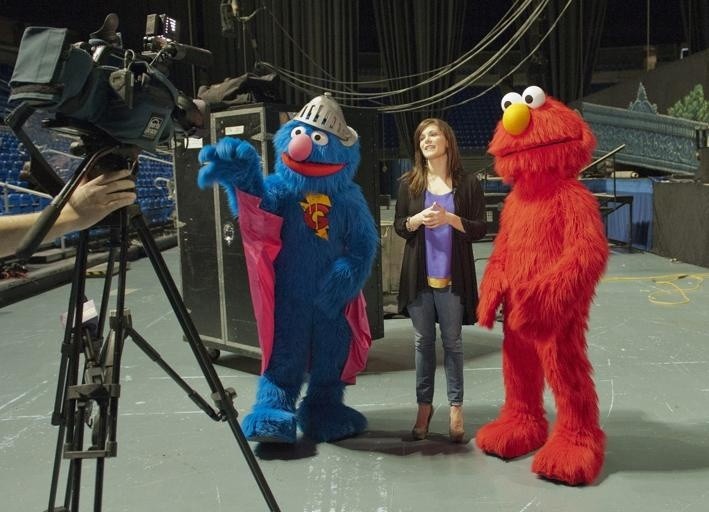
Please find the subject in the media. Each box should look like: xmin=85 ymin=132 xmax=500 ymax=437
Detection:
xmin=406 ymin=215 xmax=414 ymax=233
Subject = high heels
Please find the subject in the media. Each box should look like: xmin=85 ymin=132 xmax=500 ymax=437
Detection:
xmin=448 ymin=406 xmax=465 ymax=442
xmin=412 ymin=403 xmax=435 ymax=440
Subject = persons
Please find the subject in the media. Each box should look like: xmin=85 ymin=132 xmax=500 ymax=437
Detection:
xmin=0 ymin=168 xmax=138 ymax=259
xmin=393 ymin=118 xmax=488 ymax=445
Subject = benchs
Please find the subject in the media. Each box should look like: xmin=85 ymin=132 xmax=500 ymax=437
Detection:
xmin=0 ymin=124 xmax=175 ymax=260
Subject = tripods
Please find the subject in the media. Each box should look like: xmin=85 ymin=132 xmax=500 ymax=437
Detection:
xmin=15 ymin=143 xmax=281 ymax=511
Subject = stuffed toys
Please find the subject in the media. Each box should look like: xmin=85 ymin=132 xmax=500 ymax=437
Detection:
xmin=196 ymin=92 xmax=380 ymax=447
xmin=472 ymin=85 xmax=611 ymax=486
